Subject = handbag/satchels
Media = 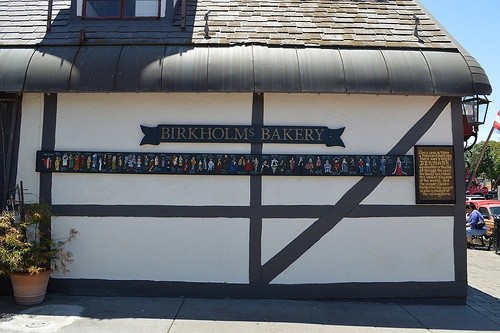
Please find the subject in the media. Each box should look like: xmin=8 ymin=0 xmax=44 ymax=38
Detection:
xmin=476 ymin=217 xmax=485 ymax=229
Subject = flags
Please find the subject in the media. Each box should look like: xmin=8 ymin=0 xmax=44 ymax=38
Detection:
xmin=493 ymin=110 xmax=500 ymax=131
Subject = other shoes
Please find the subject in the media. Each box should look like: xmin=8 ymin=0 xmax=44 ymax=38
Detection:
xmin=467 ymin=242 xmax=475 ymax=249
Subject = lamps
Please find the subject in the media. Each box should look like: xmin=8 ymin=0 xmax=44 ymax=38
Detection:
xmin=462 ymin=95 xmax=492 ymax=158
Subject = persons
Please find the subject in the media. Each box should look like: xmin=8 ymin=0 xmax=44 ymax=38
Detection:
xmin=466 ymin=202 xmax=487 ymax=247
xmin=41 ymin=153 xmax=406 ymax=176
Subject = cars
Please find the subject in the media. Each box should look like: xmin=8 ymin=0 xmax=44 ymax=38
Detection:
xmin=477 ymin=205 xmax=500 ymax=236
xmin=466 ymin=194 xmax=486 ymax=202
xmin=466 ymin=199 xmax=500 ymax=214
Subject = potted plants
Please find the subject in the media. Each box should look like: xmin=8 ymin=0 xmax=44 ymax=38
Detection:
xmin=0 ymin=181 xmax=78 ymax=306
